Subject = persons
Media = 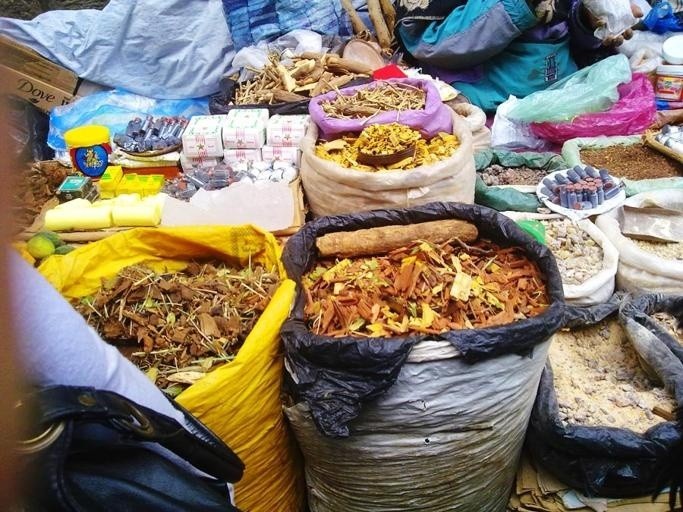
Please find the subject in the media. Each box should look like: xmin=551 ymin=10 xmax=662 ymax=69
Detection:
xmin=395 ymin=1 xmax=644 ymax=130
xmin=0 ymin=235 xmax=237 ymax=511
